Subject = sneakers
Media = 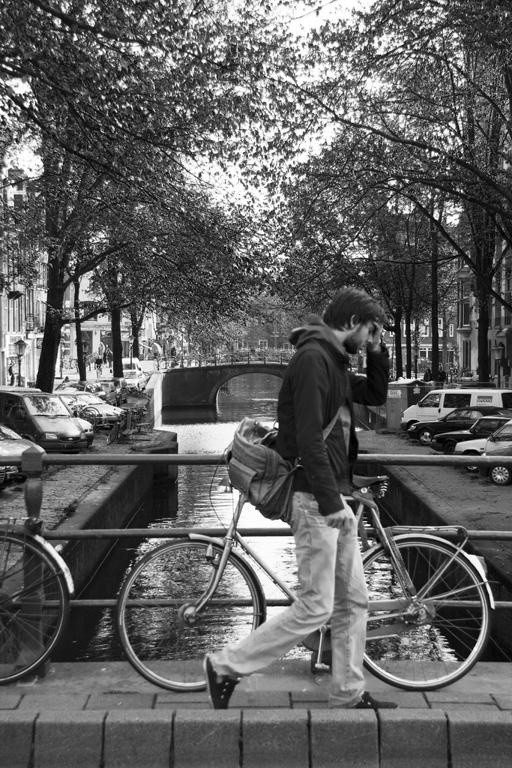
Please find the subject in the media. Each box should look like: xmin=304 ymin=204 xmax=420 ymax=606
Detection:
xmin=203 ymin=654 xmax=240 ymax=710
xmin=348 ymin=691 xmax=399 ymax=709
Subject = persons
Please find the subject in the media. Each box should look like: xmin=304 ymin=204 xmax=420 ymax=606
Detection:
xmin=251 ymin=349 xmax=263 ymax=360
xmin=104 ymin=348 xmax=113 ymax=368
xmin=94 ymin=355 xmax=103 ymax=375
xmin=424 ymin=368 xmax=432 ymax=381
xmin=203 ymin=288 xmax=398 ymax=709
xmin=9 ymin=360 xmax=14 ymax=386
xmin=453 ymin=366 xmax=458 ymax=375
xmin=439 ymin=363 xmax=443 ymax=372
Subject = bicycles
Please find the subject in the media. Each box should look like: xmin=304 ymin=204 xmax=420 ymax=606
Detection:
xmin=0 ymin=467 xmax=76 ymax=691
xmin=110 ymin=472 xmax=497 ymax=697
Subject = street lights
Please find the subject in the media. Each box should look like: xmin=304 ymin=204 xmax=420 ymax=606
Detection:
xmin=412 ymin=345 xmax=420 ymax=378
xmin=127 ymin=335 xmax=136 ymax=369
xmin=13 ymin=337 xmax=29 ymax=386
xmin=492 ymin=339 xmax=504 ymax=389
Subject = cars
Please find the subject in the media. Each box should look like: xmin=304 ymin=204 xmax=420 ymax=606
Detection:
xmin=0 ymin=348 xmax=168 ymax=454
xmin=399 ymin=385 xmax=512 ymax=487
xmin=0 ymin=422 xmax=49 ymax=489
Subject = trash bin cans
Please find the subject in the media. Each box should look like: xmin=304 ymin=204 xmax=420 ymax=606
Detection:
xmin=387 ymin=380 xmax=432 ymax=433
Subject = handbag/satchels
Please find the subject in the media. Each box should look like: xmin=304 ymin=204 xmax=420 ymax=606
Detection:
xmin=228 ymin=416 xmax=293 ymax=521
